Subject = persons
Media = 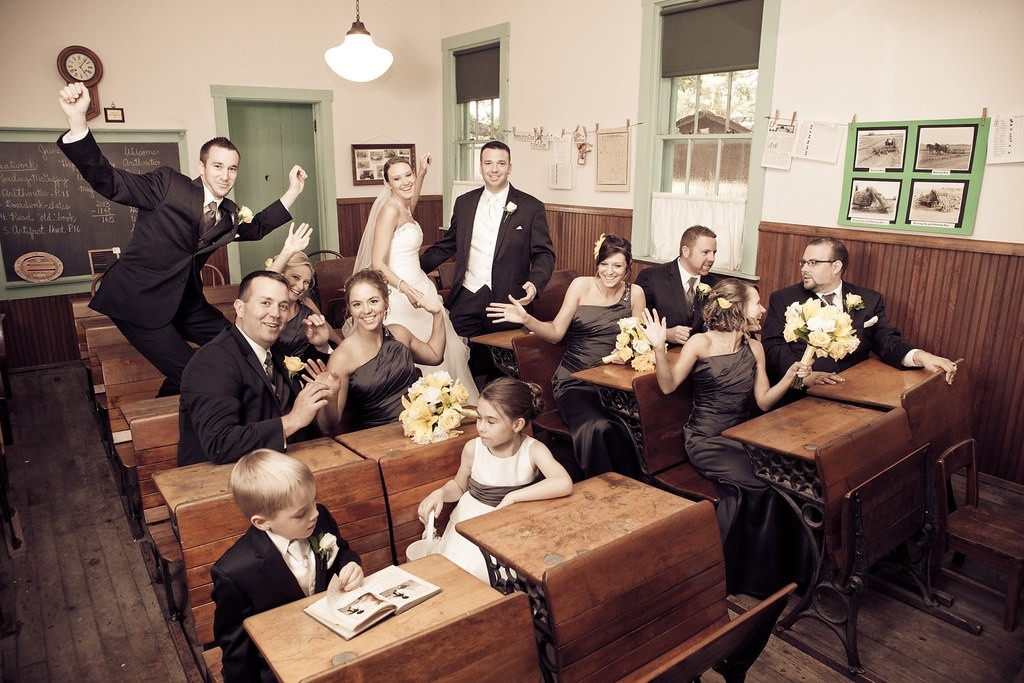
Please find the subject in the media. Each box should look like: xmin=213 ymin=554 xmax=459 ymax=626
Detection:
xmin=752 ymin=236 xmax=958 ymax=410
xmin=636 ymin=278 xmax=821 ymax=598
xmin=209 ymin=449 xmax=365 ymax=683
xmin=634 ymin=224 xmax=718 ymax=351
xmin=56 ymin=80 xmax=309 ymax=400
xmin=263 ymin=222 xmax=344 ymax=359
xmin=298 ymin=269 xmax=445 ymax=433
xmin=419 ymin=141 xmax=556 ymax=345
xmin=175 ymin=270 xmax=331 ymax=466
xmin=415 ymin=375 xmax=573 ymax=588
xmin=485 ymin=233 xmax=646 ymax=480
xmin=353 ymin=152 xmax=481 ymax=410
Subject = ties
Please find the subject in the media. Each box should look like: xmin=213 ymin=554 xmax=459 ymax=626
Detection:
xmin=822 ymin=293 xmax=835 ymax=306
xmin=488 ymin=196 xmax=497 ymax=219
xmin=264 ymin=352 xmax=275 ymax=387
xmin=201 ymin=201 xmax=217 ymax=236
xmin=687 ymin=277 xmax=698 ymax=306
xmin=288 ymin=539 xmax=310 ymax=572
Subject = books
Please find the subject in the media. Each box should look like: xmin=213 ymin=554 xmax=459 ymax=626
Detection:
xmin=302 ymin=565 xmax=442 ymax=641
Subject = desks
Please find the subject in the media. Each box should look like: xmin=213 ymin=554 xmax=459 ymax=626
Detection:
xmin=805 ymin=358 xmax=931 ymax=408
xmin=150 ymin=437 xmax=363 ymax=550
xmin=243 ymin=554 xmax=504 ymax=683
xmin=721 ymin=397 xmax=983 ymax=674
xmin=570 ymin=346 xmax=682 ymax=483
xmin=455 ymin=472 xmax=698 ymax=683
xmin=335 ymin=423 xmax=476 ymax=461
xmin=470 ymin=329 xmax=526 ymax=379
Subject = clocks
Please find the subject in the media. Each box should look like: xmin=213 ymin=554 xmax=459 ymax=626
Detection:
xmin=56 ymin=45 xmax=104 ymax=121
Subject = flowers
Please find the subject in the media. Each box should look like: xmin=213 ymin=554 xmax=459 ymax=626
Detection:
xmin=601 ymin=317 xmax=669 ymax=373
xmin=783 ymin=298 xmax=861 ymax=390
xmin=237 ymin=206 xmax=255 ymax=225
xmin=283 ymin=356 xmax=305 ymax=381
xmin=502 ymin=201 xmax=518 ymax=223
xmin=844 ymin=292 xmax=865 ymax=313
xmin=399 ymin=371 xmax=478 ymax=446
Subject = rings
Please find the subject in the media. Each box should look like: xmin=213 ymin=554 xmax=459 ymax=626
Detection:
xmin=953 ymin=364 xmax=955 ymax=367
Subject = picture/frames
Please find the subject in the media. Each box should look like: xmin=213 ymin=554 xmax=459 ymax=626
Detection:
xmin=104 ymin=107 xmax=125 ymax=123
xmin=351 ymin=144 xmax=416 ymax=186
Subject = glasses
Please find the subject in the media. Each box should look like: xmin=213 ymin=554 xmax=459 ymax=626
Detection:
xmin=798 ymin=259 xmax=837 ymax=267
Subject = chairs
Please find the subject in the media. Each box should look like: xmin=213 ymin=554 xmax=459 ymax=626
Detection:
xmin=69 ymin=264 xmax=1024 ymax=683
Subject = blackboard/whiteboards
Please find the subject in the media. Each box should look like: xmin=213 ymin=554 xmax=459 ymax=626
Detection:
xmin=1 ymin=127 xmax=193 ymax=300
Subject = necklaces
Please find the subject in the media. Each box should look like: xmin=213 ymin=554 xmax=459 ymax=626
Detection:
xmin=390 ymin=193 xmax=412 ymax=217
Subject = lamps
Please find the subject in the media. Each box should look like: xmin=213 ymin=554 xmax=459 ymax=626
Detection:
xmin=323 ymin=0 xmax=393 ymax=83
